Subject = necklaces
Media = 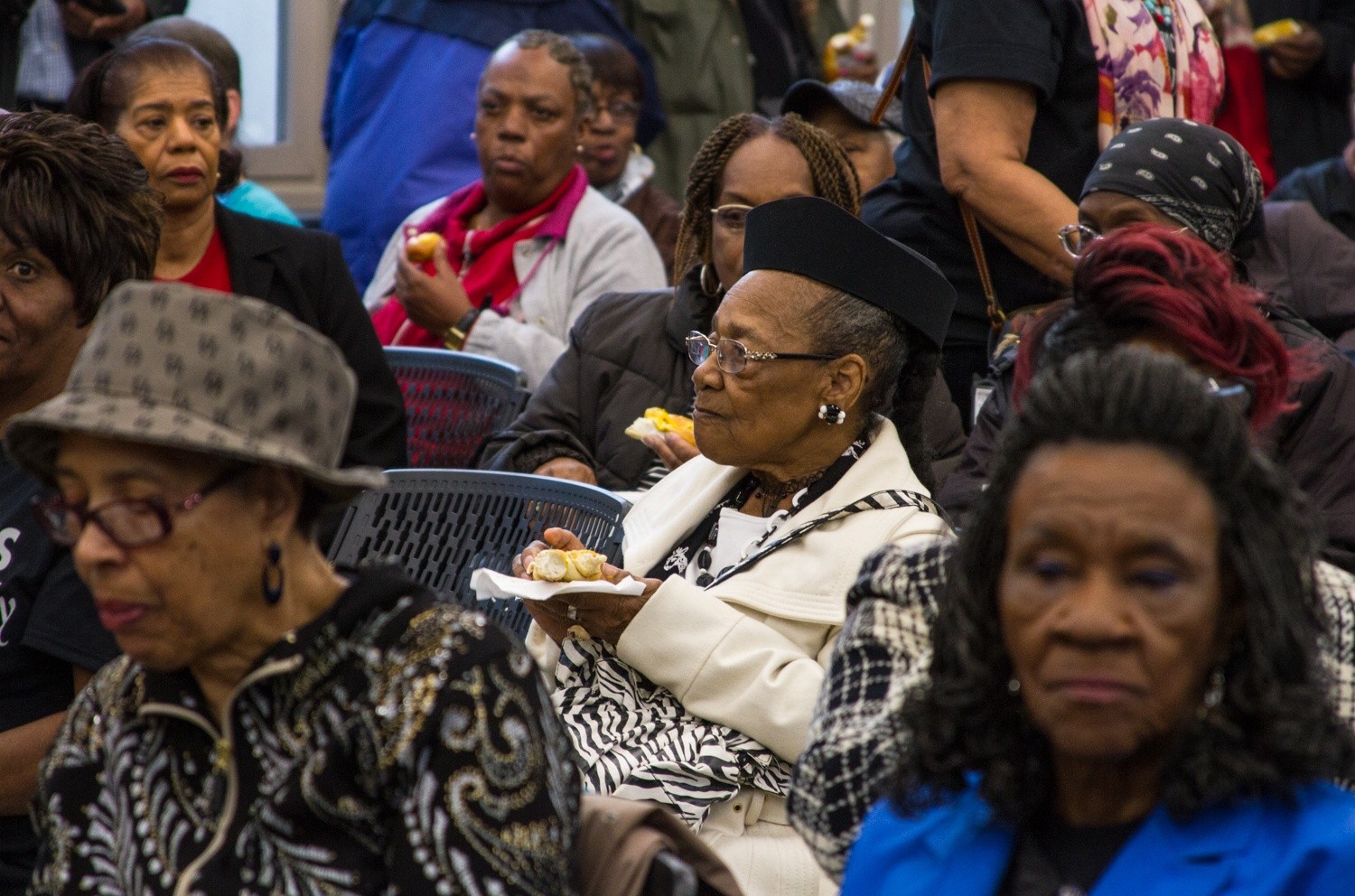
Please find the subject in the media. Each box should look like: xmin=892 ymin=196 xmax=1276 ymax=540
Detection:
xmin=698 ymin=465 xmax=825 ymax=588
xmin=746 ymin=468 xmax=821 ymax=516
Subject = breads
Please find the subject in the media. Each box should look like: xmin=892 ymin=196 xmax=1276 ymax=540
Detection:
xmin=821 ymin=13 xmax=877 ymax=84
xmin=526 ymin=549 xmax=607 ymax=582
xmin=625 ymin=407 xmax=696 ymax=446
xmin=1252 ymin=18 xmax=1303 ymax=47
xmin=406 ymin=232 xmax=444 ymax=262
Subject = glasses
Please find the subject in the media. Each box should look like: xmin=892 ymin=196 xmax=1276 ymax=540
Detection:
xmin=684 ymin=330 xmax=870 ymax=385
xmin=708 ymin=203 xmax=754 ymax=233
xmin=30 ymin=459 xmax=257 ymax=548
xmin=583 ymin=97 xmax=642 ymax=118
xmin=1055 ymin=222 xmax=1190 ymax=259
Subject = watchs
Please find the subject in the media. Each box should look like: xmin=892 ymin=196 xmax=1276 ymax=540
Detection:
xmin=444 ymin=308 xmax=480 ymax=352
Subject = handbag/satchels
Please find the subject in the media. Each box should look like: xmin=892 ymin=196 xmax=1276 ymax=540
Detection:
xmin=986 ymin=304 xmax=1058 ymax=379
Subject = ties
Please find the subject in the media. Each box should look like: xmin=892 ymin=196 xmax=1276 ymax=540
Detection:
xmin=779 ymin=77 xmax=909 ymax=135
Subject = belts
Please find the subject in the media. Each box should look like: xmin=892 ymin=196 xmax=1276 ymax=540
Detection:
xmin=15 ymin=100 xmax=66 ymax=114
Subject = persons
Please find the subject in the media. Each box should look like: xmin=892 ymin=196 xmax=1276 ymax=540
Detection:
xmin=0 ymin=0 xmax=1355 ymax=896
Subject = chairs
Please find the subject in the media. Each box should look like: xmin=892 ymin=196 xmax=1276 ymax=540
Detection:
xmin=356 ymin=334 xmax=534 ymax=471
xmin=317 ymin=461 xmax=636 ymax=645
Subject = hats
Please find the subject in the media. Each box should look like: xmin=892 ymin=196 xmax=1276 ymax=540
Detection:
xmin=2 ymin=280 xmax=386 ymax=503
xmin=744 ymin=197 xmax=959 ymax=353
